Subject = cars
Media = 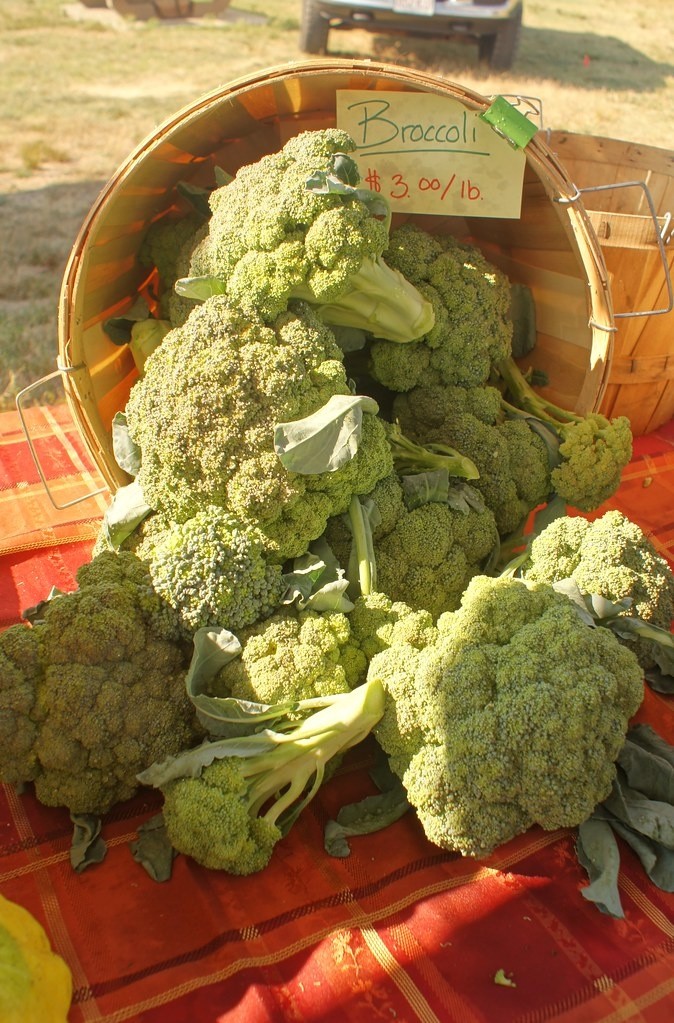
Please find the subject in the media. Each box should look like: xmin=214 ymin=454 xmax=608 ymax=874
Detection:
xmin=300 ymin=0 xmax=524 ymax=72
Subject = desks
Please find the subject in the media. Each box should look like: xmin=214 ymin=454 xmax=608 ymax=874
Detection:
xmin=0 ymin=406 xmax=674 ymax=1023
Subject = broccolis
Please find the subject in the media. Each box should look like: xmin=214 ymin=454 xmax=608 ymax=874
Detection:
xmin=0 ymin=130 xmax=671 ymax=877
